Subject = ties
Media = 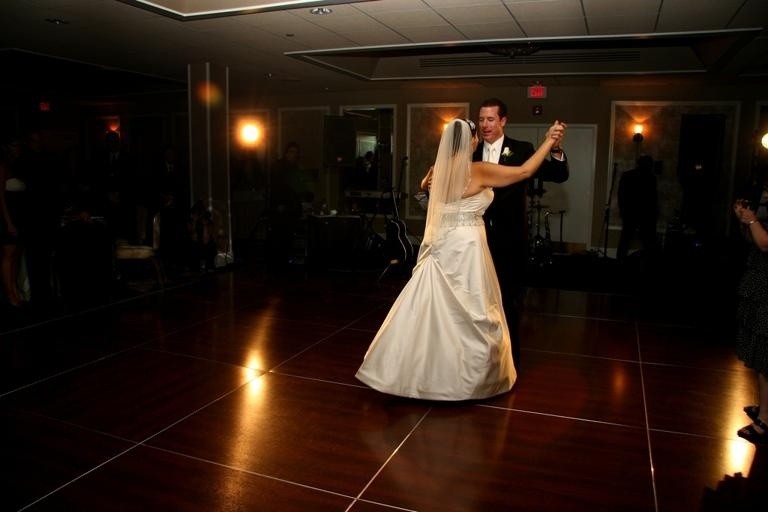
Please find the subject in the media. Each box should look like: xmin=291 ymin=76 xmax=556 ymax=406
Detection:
xmin=488 ymin=145 xmax=495 ymax=163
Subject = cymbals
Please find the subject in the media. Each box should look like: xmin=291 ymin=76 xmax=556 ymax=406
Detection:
xmin=531 ymin=204 xmax=550 ymax=209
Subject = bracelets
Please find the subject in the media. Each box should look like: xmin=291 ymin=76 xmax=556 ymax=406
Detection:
xmin=744 ymin=218 xmax=757 ymax=228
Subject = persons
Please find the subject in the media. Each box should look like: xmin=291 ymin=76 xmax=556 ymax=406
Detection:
xmin=415 ymin=98 xmax=571 ymax=370
xmin=20 ymin=129 xmax=65 ymax=307
xmin=732 ymin=182 xmax=767 ymax=439
xmin=355 ymin=117 xmax=567 ymax=404
xmin=617 ymin=155 xmax=659 ymax=253
xmin=1 ymin=133 xmax=31 ymax=307
xmin=267 ymin=141 xmax=317 ymax=230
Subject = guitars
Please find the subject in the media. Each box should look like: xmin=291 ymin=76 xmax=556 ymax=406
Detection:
xmin=390 ymin=192 xmax=413 ymax=261
xmin=380 ymin=194 xmax=406 ymax=264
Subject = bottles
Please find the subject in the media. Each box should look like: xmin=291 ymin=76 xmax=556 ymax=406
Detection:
xmin=314 ymin=202 xmax=330 ymax=215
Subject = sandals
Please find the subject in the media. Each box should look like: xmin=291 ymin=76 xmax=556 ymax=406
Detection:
xmin=744 ymin=405 xmax=760 ymax=419
xmin=738 ymin=419 xmax=768 ymax=440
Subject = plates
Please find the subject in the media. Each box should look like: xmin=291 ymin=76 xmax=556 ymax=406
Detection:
xmin=311 ymin=209 xmax=337 ymax=218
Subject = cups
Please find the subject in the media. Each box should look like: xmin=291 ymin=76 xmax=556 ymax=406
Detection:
xmin=736 ymin=199 xmax=751 ymax=210
